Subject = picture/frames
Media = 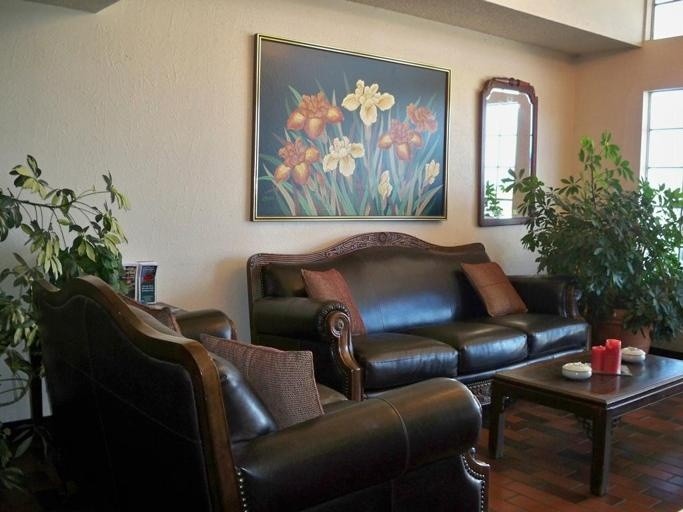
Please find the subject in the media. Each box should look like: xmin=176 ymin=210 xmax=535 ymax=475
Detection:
xmin=250 ymin=32 xmax=451 ymax=222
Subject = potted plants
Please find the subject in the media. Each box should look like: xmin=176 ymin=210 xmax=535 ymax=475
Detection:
xmin=500 ymin=131 xmax=683 ymax=355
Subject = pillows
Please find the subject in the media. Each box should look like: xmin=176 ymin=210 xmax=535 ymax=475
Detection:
xmin=301 ymin=269 xmax=366 ymax=336
xmin=459 ymin=262 xmax=528 ymax=317
xmin=200 ymin=333 xmax=324 ymax=430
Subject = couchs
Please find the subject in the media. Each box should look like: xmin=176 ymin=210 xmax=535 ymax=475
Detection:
xmin=247 ymin=231 xmax=592 ymax=407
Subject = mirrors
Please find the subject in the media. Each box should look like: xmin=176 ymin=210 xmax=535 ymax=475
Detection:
xmin=478 ymin=78 xmax=538 ymax=227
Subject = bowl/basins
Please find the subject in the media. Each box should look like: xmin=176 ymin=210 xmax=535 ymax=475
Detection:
xmin=561 ymin=361 xmax=594 ymax=381
xmin=622 ymin=346 xmax=647 ymax=363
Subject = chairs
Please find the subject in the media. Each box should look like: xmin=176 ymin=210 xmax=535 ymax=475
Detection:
xmin=32 ymin=275 xmax=491 ymax=512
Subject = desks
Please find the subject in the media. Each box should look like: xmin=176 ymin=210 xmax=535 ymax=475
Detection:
xmin=489 ymin=351 xmax=683 ymax=496
